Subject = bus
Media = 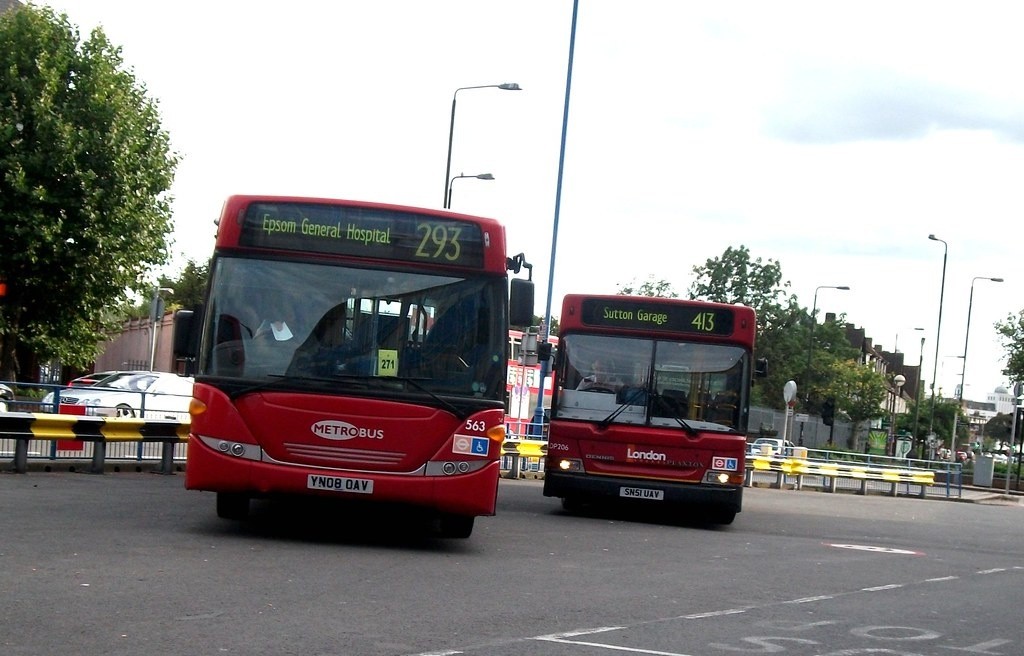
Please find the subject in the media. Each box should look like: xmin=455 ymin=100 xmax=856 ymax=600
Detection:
xmin=537 ymin=294 xmax=767 ymax=523
xmin=148 ymin=195 xmax=533 ymax=540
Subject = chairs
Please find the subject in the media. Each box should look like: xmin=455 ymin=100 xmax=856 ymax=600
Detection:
xmin=630 ymin=384 xmax=738 ymax=431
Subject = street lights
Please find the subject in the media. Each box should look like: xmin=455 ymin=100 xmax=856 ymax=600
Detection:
xmin=447 ymin=172 xmax=495 ymax=210
xmin=888 ymin=374 xmax=905 ymax=457
xmin=798 ymin=286 xmax=850 ymax=447
xmin=923 ymin=234 xmax=948 ymax=468
xmin=442 ymin=83 xmax=521 ymax=208
xmin=951 ymin=276 xmax=1004 ymax=462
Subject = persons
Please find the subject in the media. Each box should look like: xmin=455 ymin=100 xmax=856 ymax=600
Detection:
xmin=252 ymin=295 xmax=321 ymax=351
xmin=576 ymin=354 xmax=625 ymax=394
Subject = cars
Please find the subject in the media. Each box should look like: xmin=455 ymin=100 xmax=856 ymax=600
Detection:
xmin=39 ymin=370 xmax=119 ymax=414
xmin=934 ymin=444 xmax=1024 ymax=465
xmin=48 ymin=371 xmax=175 ymax=419
xmin=751 ymin=438 xmax=795 ymax=456
